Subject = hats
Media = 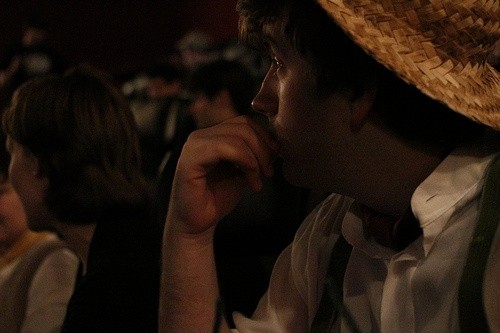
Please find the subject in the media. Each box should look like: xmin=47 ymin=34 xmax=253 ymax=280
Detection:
xmin=316 ymin=0 xmax=500 ymax=133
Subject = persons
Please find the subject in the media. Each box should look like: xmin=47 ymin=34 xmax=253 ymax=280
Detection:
xmin=158 ymin=0 xmax=500 ymax=333
xmin=0 ymin=20 xmax=319 ymax=333
